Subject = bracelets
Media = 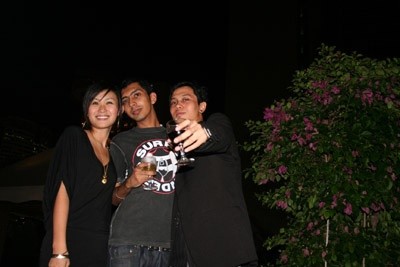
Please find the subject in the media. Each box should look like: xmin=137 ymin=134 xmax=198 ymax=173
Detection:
xmin=50 ymin=253 xmax=70 ymax=260
xmin=204 ymin=128 xmax=212 ymax=139
xmin=114 ymin=188 xmax=125 ymax=200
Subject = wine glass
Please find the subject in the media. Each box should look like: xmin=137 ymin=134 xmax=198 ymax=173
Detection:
xmin=167 ymin=119 xmax=195 ymax=166
xmin=143 ymin=158 xmax=157 ymax=189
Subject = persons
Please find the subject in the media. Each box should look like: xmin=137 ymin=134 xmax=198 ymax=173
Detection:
xmin=108 ymin=76 xmax=175 ymax=267
xmin=40 ymin=81 xmax=125 ymax=267
xmin=169 ymin=82 xmax=257 ymax=267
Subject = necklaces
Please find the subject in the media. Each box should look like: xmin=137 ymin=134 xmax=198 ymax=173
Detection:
xmin=93 ymin=138 xmax=109 ymax=184
